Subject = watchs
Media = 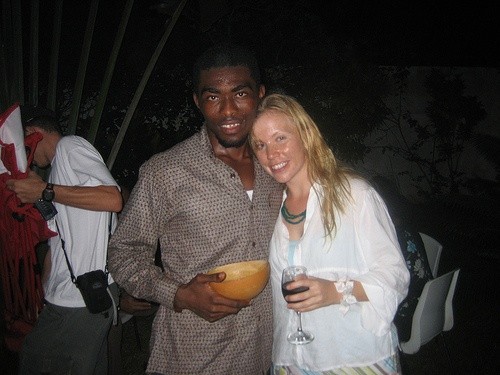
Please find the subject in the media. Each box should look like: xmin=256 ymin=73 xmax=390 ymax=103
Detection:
xmin=42 ymin=183 xmax=54 ymax=201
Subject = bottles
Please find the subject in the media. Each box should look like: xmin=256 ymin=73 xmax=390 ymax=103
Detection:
xmin=34 ymin=198 xmax=58 ymax=220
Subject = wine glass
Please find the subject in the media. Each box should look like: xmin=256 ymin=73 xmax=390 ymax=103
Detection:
xmin=281 ymin=265 xmax=315 ymax=345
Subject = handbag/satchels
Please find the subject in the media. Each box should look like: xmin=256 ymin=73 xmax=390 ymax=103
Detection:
xmin=75 ymin=269 xmax=113 ymax=318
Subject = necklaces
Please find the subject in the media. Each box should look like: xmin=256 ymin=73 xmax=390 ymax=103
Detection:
xmin=281 ymin=200 xmax=306 ymax=224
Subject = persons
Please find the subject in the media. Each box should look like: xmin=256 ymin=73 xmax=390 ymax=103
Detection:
xmin=6 ymin=105 xmax=123 ymax=375
xmin=105 ymin=43 xmax=285 ymax=375
xmin=247 ymin=92 xmax=410 ymax=375
xmin=372 ymin=175 xmax=434 ymax=342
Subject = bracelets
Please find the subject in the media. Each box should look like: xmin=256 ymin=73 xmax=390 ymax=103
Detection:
xmin=334 ymin=281 xmax=358 ymax=306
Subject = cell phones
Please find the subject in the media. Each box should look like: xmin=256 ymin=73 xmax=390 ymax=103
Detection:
xmin=35 ymin=198 xmax=57 ymax=221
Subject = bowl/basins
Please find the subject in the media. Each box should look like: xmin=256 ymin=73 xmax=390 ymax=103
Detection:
xmin=207 ymin=260 xmax=269 ymax=303
